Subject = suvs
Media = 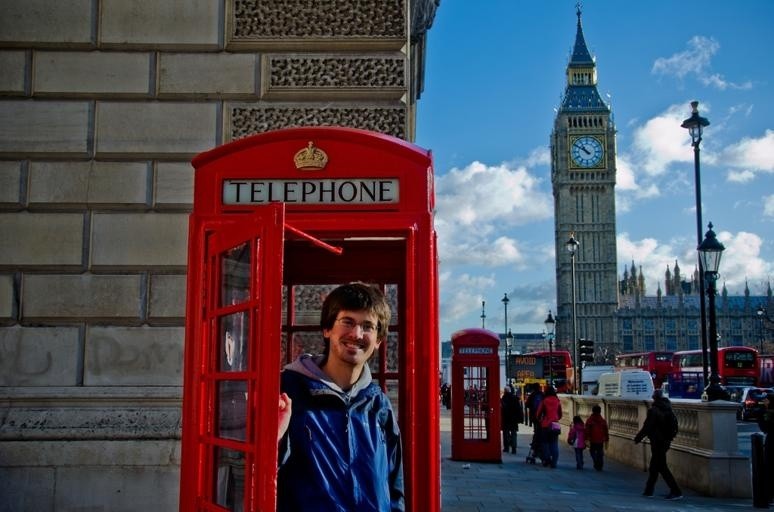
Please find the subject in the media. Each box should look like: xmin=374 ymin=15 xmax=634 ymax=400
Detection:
xmin=734 ymin=388 xmax=774 ymax=421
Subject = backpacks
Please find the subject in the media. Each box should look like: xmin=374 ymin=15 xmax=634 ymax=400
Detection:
xmin=662 ymin=413 xmax=678 ymax=440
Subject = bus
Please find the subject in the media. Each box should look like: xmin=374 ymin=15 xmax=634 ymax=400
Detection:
xmin=670 ymin=345 xmax=760 ymax=389
xmin=504 ymin=349 xmax=573 ymax=393
xmin=615 ymin=351 xmax=675 ymax=389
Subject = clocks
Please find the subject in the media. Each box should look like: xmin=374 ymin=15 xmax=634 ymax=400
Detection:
xmin=567 ymin=133 xmax=608 ymax=171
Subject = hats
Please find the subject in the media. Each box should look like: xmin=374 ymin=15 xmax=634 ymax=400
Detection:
xmin=652 ymin=389 xmax=669 ymax=399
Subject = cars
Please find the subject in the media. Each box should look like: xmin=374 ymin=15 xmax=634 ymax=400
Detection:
xmin=723 ymin=385 xmax=759 ymax=403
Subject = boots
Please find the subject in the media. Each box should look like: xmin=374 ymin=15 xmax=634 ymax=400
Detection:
xmin=663 ymin=477 xmax=684 ymax=501
xmin=642 ymin=476 xmax=658 ymax=498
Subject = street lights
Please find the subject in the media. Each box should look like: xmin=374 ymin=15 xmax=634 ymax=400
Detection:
xmin=501 ymin=291 xmax=511 ymax=377
xmin=565 ymin=230 xmax=581 ymax=395
xmin=756 ymin=305 xmax=767 ymax=356
xmin=716 ymin=331 xmax=722 ymax=349
xmin=479 ymin=300 xmax=488 ymax=327
xmin=680 ymin=97 xmax=713 ymax=386
xmin=544 ymin=309 xmax=556 ymax=389
xmin=505 ymin=327 xmax=515 ymax=387
xmin=697 ymin=219 xmax=730 ymax=398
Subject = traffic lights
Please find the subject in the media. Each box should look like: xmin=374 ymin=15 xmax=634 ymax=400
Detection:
xmin=578 ymin=337 xmax=595 ymax=369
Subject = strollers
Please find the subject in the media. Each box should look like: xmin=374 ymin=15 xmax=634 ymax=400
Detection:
xmin=525 ymin=416 xmax=551 ymax=466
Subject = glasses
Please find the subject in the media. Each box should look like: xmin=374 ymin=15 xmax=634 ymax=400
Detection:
xmin=336 ymin=317 xmax=381 ymax=331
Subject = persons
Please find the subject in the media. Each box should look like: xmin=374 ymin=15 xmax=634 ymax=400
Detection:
xmin=441 ymin=383 xmax=609 ymax=472
xmin=682 ymin=384 xmax=701 ymax=398
xmin=660 ymin=377 xmax=670 ymax=399
xmin=756 ymin=393 xmax=774 ymax=506
xmin=705 ymin=374 xmax=731 ymax=402
xmin=635 ymin=389 xmax=684 ymax=501
xmin=277 ymin=285 xmax=407 ymax=512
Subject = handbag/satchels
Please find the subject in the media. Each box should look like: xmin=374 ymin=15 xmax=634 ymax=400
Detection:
xmin=551 ymin=421 xmax=561 ymax=435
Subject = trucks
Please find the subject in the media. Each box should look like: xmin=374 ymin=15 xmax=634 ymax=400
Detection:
xmin=591 ymin=370 xmax=656 ymax=401
xmin=577 ymin=364 xmax=616 ymax=398
